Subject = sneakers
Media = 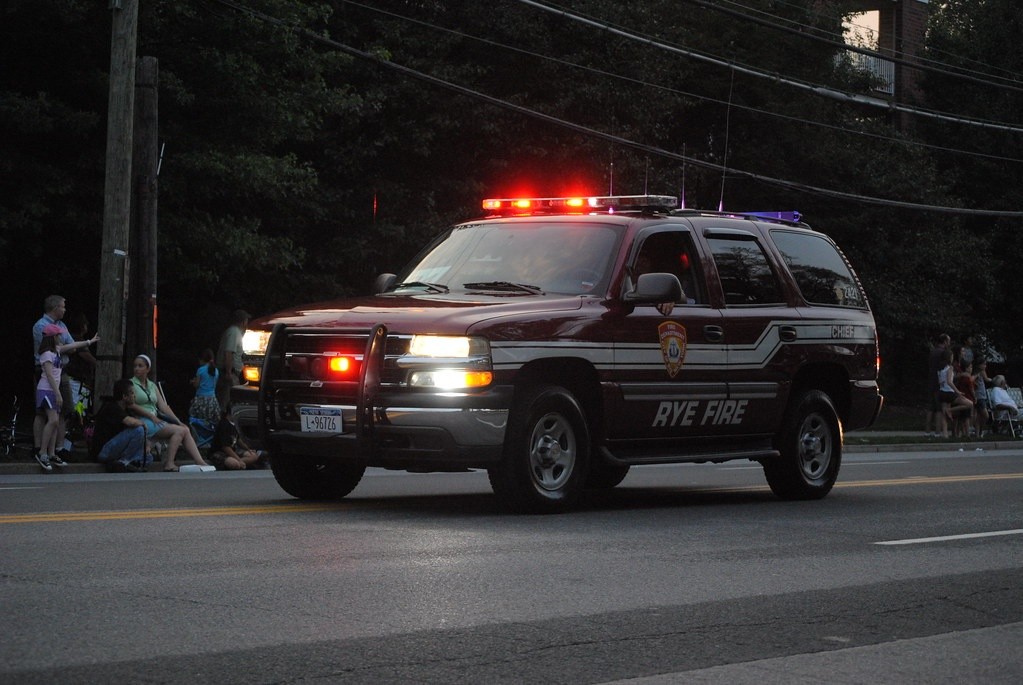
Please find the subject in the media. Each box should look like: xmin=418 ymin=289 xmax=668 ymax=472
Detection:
xmin=49 ymin=454 xmax=67 ymax=466
xmin=35 ymin=452 xmax=52 ymax=470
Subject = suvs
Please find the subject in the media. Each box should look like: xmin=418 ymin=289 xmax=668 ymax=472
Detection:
xmin=227 ymin=194 xmax=884 ymax=517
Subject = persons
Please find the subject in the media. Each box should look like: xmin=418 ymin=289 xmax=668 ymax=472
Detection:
xmin=35 ymin=325 xmax=101 ymax=470
xmin=208 ymin=400 xmax=258 ymax=470
xmin=924 ymin=332 xmax=1023 ymax=440
xmin=129 ymin=354 xmax=211 ymax=472
xmin=91 ymin=379 xmax=153 ymax=472
xmin=30 ymin=295 xmax=77 ymax=461
xmin=216 ymin=310 xmax=251 ymax=419
xmin=597 ymin=244 xmax=689 ymax=315
xmin=189 ymin=349 xmax=222 ymax=426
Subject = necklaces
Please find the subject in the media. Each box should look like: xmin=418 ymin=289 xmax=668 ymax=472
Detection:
xmin=139 ymin=386 xmax=152 ymax=402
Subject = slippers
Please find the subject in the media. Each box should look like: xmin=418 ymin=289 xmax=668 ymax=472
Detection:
xmin=164 ymin=466 xmax=179 ymax=471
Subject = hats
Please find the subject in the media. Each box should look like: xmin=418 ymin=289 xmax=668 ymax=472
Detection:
xmin=42 ymin=323 xmax=68 ymax=337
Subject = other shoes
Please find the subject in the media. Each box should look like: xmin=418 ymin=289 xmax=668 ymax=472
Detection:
xmin=114 ymin=461 xmax=140 ymax=472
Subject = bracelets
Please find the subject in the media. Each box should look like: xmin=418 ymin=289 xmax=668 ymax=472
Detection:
xmin=87 ymin=340 xmax=90 ymax=346
